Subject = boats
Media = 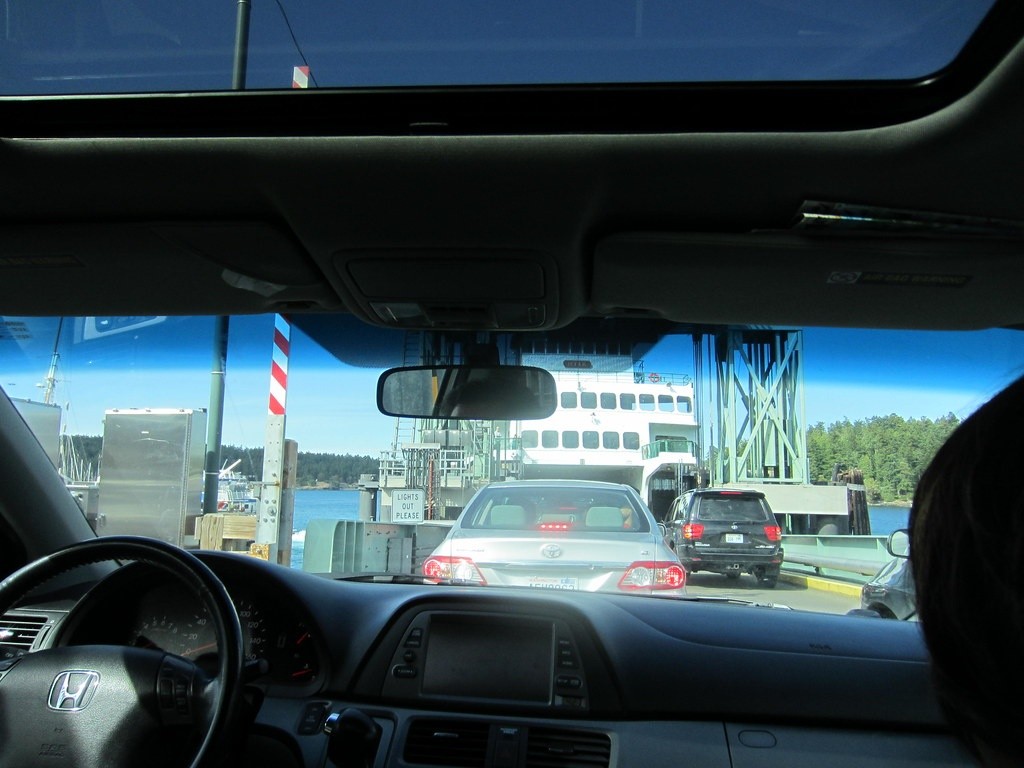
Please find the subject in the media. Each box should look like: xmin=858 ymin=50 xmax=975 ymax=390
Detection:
xmin=357 ymin=343 xmax=712 ymax=522
xmin=197 ymin=455 xmax=261 ymax=514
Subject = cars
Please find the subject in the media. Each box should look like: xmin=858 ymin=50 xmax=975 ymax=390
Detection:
xmin=857 ymin=526 xmax=918 ymax=624
xmin=421 ymin=477 xmax=687 ymax=600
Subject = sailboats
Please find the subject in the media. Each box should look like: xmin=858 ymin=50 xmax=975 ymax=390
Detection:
xmin=34 ymin=318 xmax=102 ymax=521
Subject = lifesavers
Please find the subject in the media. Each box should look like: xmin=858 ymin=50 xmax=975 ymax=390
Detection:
xmin=650 ymin=373 xmax=660 ymax=382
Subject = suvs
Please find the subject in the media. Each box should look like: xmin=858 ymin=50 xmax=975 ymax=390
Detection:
xmin=659 ymin=484 xmax=785 ymax=588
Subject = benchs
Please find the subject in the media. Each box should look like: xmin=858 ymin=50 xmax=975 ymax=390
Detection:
xmin=490 ymin=504 xmax=626 ymax=530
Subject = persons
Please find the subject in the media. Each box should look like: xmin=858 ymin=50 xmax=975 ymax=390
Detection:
xmin=908 ymin=373 xmax=1024 ymax=768
